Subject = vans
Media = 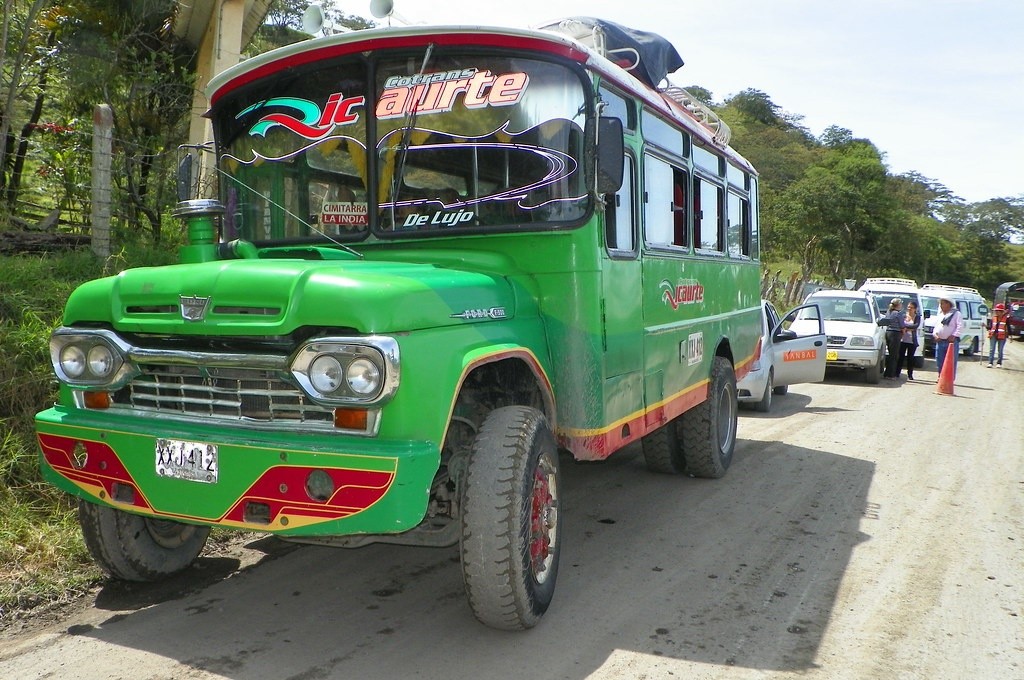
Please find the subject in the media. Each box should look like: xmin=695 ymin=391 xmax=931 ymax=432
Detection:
xmin=916 ymin=283 xmax=988 ymax=356
xmin=852 ymin=277 xmax=930 ymax=368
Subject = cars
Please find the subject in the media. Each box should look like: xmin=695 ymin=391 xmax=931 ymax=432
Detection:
xmin=733 ymin=298 xmax=828 ymax=412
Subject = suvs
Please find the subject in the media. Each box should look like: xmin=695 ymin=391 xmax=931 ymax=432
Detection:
xmin=788 ymin=288 xmax=890 ymax=384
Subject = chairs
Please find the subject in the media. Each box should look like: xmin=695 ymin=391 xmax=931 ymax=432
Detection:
xmin=846 ymin=301 xmax=870 ymax=320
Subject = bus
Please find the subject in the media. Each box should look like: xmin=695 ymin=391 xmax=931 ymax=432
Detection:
xmin=33 ymin=20 xmax=765 ymax=635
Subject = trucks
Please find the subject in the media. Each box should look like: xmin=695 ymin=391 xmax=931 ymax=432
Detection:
xmin=989 ymin=281 xmax=1023 ymax=341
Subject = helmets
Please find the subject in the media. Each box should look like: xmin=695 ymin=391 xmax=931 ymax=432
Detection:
xmin=992 ymin=304 xmax=1005 ymax=311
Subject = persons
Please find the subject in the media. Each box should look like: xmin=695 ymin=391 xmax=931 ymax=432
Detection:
xmin=335 ymin=178 xmax=526 ymax=241
xmin=982 ymin=303 xmax=1013 ymax=368
xmin=932 ymin=296 xmax=962 ymax=384
xmin=895 ymin=302 xmax=921 ymax=380
xmin=883 ymin=298 xmax=906 ymax=381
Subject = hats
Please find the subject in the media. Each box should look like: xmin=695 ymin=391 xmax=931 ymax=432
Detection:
xmin=938 ymin=296 xmax=956 ymax=307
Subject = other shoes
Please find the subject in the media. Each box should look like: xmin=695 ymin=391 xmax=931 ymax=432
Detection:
xmin=896 ymin=368 xmax=901 ymax=377
xmin=997 ymin=364 xmax=1002 ymax=367
xmin=885 ymin=376 xmax=896 ymax=381
xmin=907 ymin=370 xmax=913 ymax=380
xmin=988 ymin=364 xmax=994 ymax=368
